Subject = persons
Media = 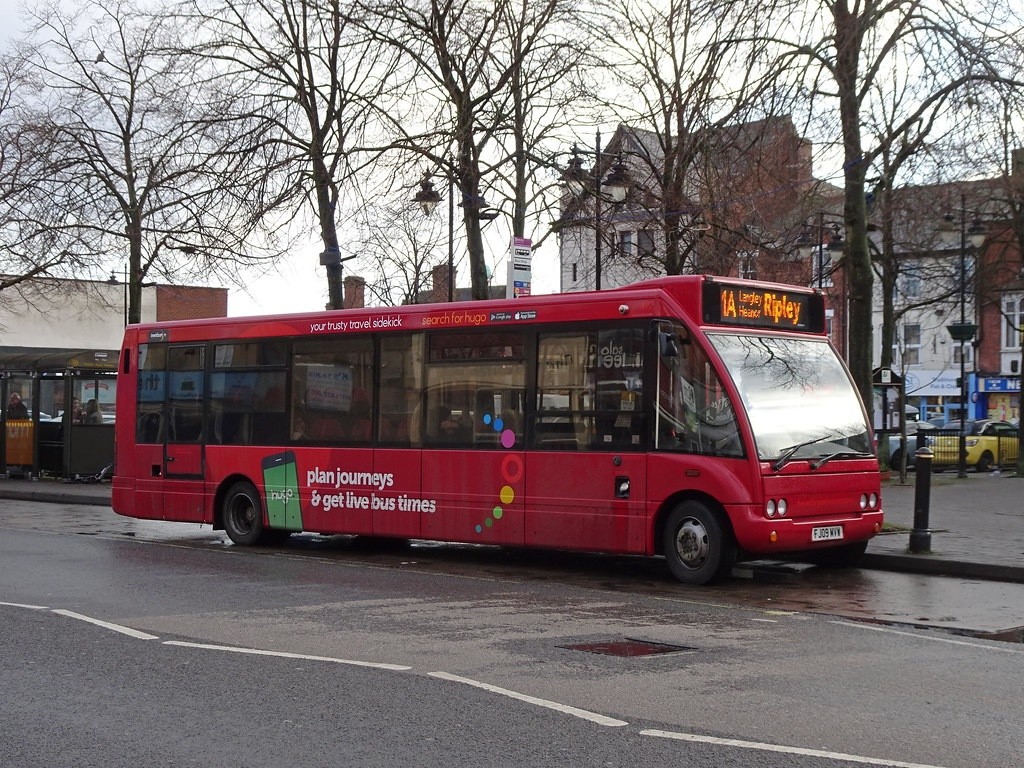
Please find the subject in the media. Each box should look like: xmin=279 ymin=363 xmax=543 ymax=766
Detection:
xmin=62 ymin=397 xmax=84 ymax=425
xmin=7 ymin=392 xmax=30 ymax=420
xmin=1008 ymin=406 xmax=1019 ymax=426
xmin=86 ymin=398 xmax=104 ymax=425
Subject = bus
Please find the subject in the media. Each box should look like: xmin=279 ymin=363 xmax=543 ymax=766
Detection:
xmin=109 ymin=273 xmax=885 ymax=588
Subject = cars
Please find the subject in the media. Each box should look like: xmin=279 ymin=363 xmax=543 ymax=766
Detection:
xmin=928 ymin=418 xmax=1020 ymax=472
xmin=873 ymin=420 xmax=938 ymax=472
